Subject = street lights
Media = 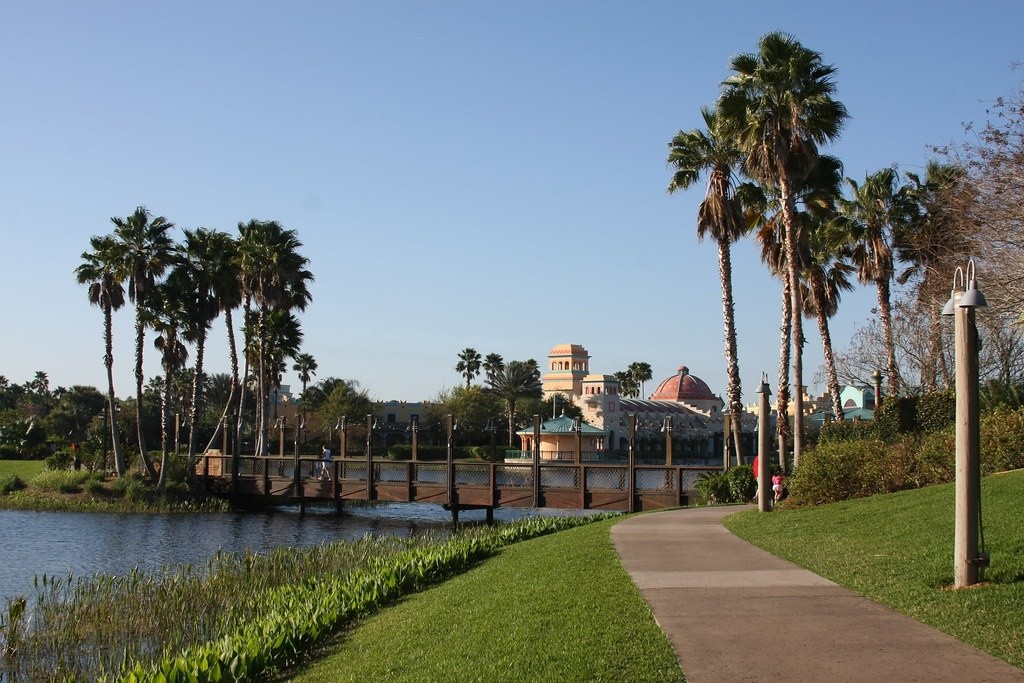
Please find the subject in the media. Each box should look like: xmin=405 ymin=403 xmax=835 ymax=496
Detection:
xmin=102 ymin=399 xmax=122 ymax=471
xmin=172 ymin=408 xmax=743 ymax=501
xmin=942 ymin=257 xmax=989 ymax=589
xmin=755 ymin=371 xmax=772 ymax=511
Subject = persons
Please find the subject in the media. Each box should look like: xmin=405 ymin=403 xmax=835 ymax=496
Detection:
xmin=72 ymin=440 xmax=82 ymax=471
xmin=752 ymin=455 xmax=758 ymax=504
xmin=317 ymin=444 xmax=332 ymax=482
xmin=771 ymin=470 xmax=785 ymax=506
